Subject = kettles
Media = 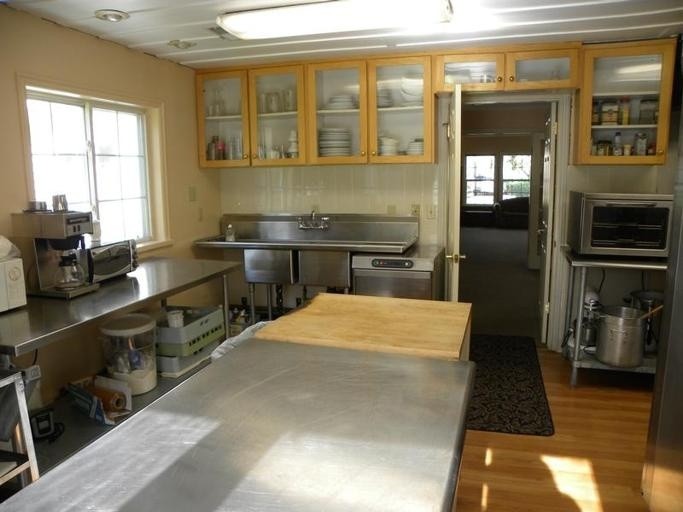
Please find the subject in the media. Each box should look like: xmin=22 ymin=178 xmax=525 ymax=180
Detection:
xmin=583 ymin=290 xmax=600 ymax=317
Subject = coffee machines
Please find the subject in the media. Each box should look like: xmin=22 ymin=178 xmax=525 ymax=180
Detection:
xmin=11 ymin=210 xmax=99 ymax=300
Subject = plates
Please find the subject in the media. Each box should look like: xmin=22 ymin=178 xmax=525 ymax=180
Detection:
xmin=377 ymin=136 xmax=399 ymax=156
xmin=405 ymin=142 xmax=424 ymax=155
xmin=318 ymin=127 xmax=353 ymax=157
xmin=398 ymin=75 xmax=423 ymax=105
xmin=317 ymin=95 xmax=358 ymax=110
xmin=376 ymin=88 xmax=393 ymax=108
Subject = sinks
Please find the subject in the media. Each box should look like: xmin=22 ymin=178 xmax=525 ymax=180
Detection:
xmin=297 ymin=240 xmax=351 ymax=288
xmin=243 ymin=237 xmax=297 ymax=285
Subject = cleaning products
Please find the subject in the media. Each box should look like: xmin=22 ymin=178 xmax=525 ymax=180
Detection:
xmin=224 ymin=224 xmax=236 ymax=242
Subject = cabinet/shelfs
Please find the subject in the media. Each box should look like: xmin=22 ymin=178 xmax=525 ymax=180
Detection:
xmin=432 ymin=49 xmax=579 ymax=93
xmin=559 ymin=251 xmax=667 ymax=389
xmin=195 ymin=64 xmax=305 ymax=167
xmin=306 ymin=54 xmax=431 ymax=164
xmin=579 ymin=44 xmax=665 ymax=164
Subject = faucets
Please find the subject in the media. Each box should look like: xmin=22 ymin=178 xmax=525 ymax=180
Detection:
xmin=311 ymin=210 xmax=317 ymax=226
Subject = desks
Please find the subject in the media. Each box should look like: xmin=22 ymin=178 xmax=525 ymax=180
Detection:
xmin=0 ymin=256 xmax=241 ymax=489
xmin=254 ymin=292 xmax=472 ymax=359
xmin=0 ymin=338 xmax=476 ymax=512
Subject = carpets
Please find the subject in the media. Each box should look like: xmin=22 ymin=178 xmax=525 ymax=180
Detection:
xmin=464 ymin=334 xmax=554 ymax=436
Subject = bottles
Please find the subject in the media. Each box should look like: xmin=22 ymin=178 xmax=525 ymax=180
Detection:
xmin=223 ymin=224 xmax=235 ymax=241
xmin=11 ymin=422 xmax=26 ymax=455
xmin=17 ymin=470 xmax=29 ymax=488
xmin=204 ymin=133 xmax=242 ymax=159
xmin=591 ymin=131 xmax=647 ymax=155
xmin=227 ymin=306 xmax=246 ymax=322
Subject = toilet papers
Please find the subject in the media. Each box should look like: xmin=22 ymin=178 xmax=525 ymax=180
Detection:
xmin=84 ymin=385 xmax=127 ymax=412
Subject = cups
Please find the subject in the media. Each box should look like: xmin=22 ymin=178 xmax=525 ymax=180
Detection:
xmin=52 ymin=194 xmax=67 ymax=212
xmin=203 ymin=86 xmax=225 ymax=116
xmin=167 ymin=310 xmax=184 ymax=327
xmin=257 ymin=87 xmax=296 ymax=114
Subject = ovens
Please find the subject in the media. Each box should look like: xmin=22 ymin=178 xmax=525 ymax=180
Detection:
xmin=566 ymin=188 xmax=674 ymax=259
xmin=352 ymin=269 xmax=431 ymax=298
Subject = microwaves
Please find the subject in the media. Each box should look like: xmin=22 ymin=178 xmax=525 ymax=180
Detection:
xmin=78 ymin=238 xmax=138 ymax=283
xmin=0 ymin=257 xmax=28 ymax=315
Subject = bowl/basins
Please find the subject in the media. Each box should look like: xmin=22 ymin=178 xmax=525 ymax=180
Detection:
xmin=574 ymin=317 xmax=603 ymax=347
xmin=285 ymin=130 xmax=296 ymax=152
